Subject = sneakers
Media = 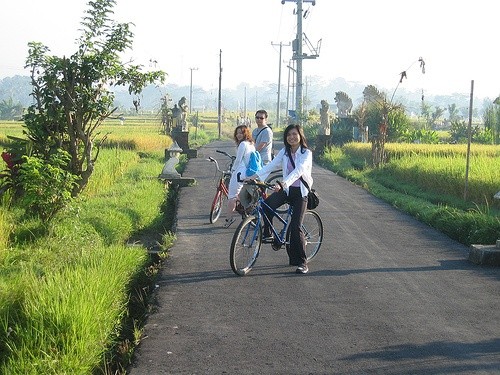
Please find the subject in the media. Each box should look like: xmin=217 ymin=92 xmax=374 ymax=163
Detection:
xmin=255 ymin=233 xmax=273 ymax=241
xmin=296 ymin=263 xmax=308 ymax=274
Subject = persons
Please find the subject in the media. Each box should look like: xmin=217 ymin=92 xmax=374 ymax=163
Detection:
xmin=223 ymin=125 xmax=258 ymax=229
xmin=251 ymin=110 xmax=273 ymax=200
xmin=242 ymin=125 xmax=314 ymax=273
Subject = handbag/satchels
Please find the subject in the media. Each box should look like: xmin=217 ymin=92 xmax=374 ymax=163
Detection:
xmin=238 ymin=184 xmax=258 ymax=212
xmin=307 ymin=189 xmax=320 ymax=210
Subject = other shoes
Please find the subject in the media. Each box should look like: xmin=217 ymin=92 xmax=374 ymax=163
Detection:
xmin=224 ymin=215 xmax=236 ymax=229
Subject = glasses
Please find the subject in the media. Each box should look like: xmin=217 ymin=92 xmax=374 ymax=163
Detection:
xmin=256 ymin=116 xmax=265 ymax=119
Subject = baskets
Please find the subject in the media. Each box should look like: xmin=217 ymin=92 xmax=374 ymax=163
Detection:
xmin=235 ymin=183 xmax=261 ymax=213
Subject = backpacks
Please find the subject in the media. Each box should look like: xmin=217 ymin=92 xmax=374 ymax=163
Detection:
xmin=242 ymin=151 xmax=263 ymax=177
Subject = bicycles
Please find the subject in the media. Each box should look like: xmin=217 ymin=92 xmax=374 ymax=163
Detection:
xmin=210 ymin=156 xmax=268 ymax=229
xmin=229 ymin=172 xmax=325 ymax=275
xmin=215 ymin=149 xmax=274 ymax=194
